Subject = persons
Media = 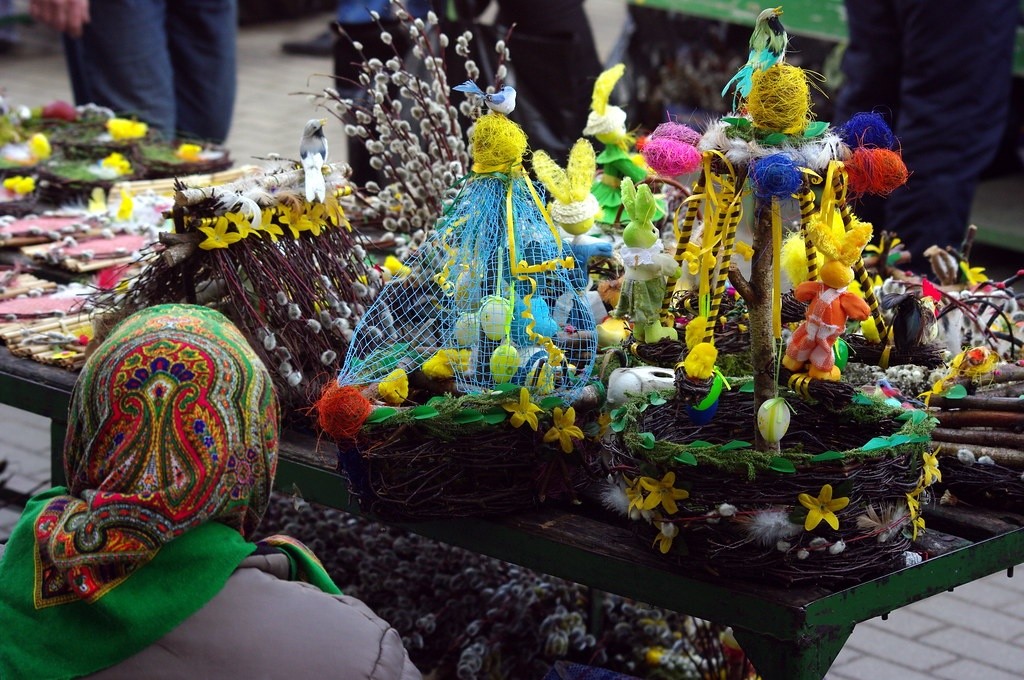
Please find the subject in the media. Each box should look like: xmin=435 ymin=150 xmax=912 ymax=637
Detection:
xmin=0 ymin=303 xmax=421 ymax=680
xmin=334 ymin=0 xmax=617 ymax=211
xmin=30 ymin=0 xmax=235 ymax=149
xmin=833 ymin=0 xmax=1017 ymax=282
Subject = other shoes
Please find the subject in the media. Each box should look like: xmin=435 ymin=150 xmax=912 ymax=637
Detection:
xmin=282 ymin=31 xmax=338 ymax=56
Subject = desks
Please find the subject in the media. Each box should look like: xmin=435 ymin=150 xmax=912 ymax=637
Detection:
xmin=2 ymin=336 xmax=1024 ymax=680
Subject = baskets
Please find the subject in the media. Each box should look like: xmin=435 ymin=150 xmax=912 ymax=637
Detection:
xmin=336 ymin=380 xmax=606 ymax=523
xmin=609 ymin=375 xmax=930 ymax=584
xmin=575 ymin=174 xmax=949 ymax=377
